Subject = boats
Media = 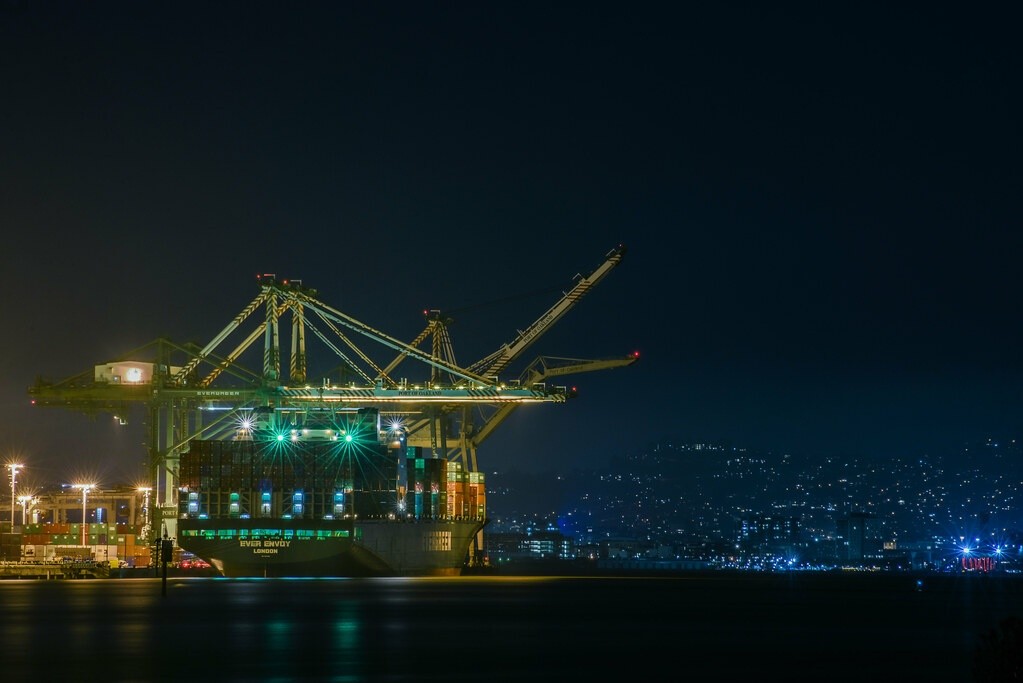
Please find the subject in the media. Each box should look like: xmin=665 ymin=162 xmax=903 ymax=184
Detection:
xmin=175 ymin=512 xmax=493 ymax=578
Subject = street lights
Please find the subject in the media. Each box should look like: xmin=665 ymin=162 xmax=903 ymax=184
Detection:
xmin=18 ymin=495 xmax=32 ymax=525
xmin=71 ymin=484 xmax=96 ymax=547
xmin=8 ymin=464 xmax=23 ymax=534
xmin=139 ymin=488 xmax=153 ymax=525
xmin=103 ymin=521 xmax=108 ymax=561
